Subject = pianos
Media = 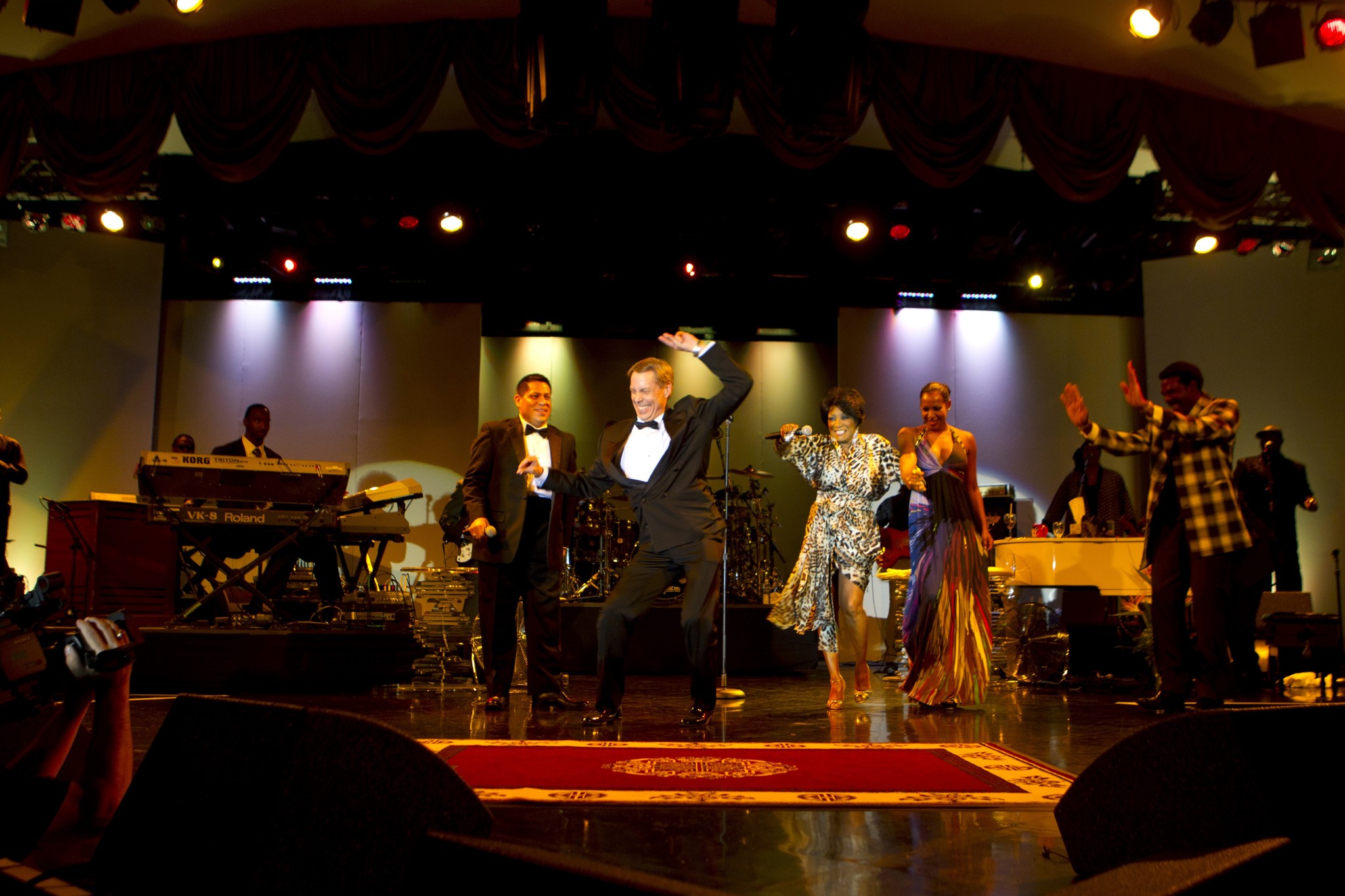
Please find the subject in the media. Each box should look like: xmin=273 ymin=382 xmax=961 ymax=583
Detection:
xmin=993 ymin=537 xmax=1199 ymax=686
xmin=137 ymin=450 xmax=425 ymax=631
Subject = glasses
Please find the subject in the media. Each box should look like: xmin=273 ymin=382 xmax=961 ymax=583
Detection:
xmin=175 ymin=444 xmax=193 ymax=450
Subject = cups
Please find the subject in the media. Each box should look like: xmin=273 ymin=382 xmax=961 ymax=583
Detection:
xmin=1031 ymin=524 xmax=1048 ymax=538
xmin=1053 ymin=522 xmax=1065 ymax=538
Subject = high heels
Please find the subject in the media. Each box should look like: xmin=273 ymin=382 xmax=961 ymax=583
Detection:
xmin=827 ymin=677 xmax=846 ymax=709
xmin=853 ymin=664 xmax=872 ymax=703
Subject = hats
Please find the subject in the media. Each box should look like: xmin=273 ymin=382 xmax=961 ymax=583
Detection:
xmin=1256 ymin=425 xmax=1281 ymax=440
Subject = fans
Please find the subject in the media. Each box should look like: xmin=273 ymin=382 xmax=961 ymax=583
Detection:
xmin=996 ymin=603 xmax=1070 ymax=687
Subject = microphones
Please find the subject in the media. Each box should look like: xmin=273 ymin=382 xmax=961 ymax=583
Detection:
xmin=764 ymin=425 xmax=812 ymax=440
xmin=40 ymin=496 xmax=68 ymax=509
xmin=1087 ymin=451 xmax=1095 ymax=458
xmin=1263 ymin=440 xmax=1275 ymax=454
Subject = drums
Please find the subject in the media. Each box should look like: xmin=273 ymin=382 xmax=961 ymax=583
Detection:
xmin=722 ymin=504 xmax=753 ymax=550
xmin=579 ymin=501 xmax=615 ymax=536
xmin=607 ymin=517 xmax=641 ymax=568
xmin=628 ymin=537 xmax=686 ymax=601
xmin=473 ymin=594 xmax=571 ymax=691
xmin=573 ymin=517 xmax=608 ymax=564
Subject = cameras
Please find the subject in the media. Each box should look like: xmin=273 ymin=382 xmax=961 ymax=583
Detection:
xmin=50 ymin=609 xmax=144 ymax=679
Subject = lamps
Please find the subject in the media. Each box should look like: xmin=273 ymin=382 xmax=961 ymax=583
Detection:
xmin=1309 ymin=0 xmax=1345 ymax=52
xmin=1187 ymin=1 xmax=1235 ymax=48
xmin=1127 ymin=0 xmax=1178 ymax=40
xmin=1247 ymin=0 xmax=1304 ymax=68
xmin=23 ymin=202 xmax=164 ymax=234
xmin=1234 ymin=228 xmax=1339 ymax=264
xmin=890 ymin=201 xmax=911 ymax=241
xmin=439 ymin=205 xmax=464 ymax=233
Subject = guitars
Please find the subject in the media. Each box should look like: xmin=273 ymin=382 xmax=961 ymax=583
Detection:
xmin=876 ymin=525 xmax=919 ymax=569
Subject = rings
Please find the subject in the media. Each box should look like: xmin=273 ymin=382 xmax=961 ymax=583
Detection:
xmin=115 ymin=629 xmax=127 ymax=640
xmin=472 ymin=533 xmax=477 ymax=537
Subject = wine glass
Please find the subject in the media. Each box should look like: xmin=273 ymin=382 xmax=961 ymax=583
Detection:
xmin=1004 ymin=513 xmax=1017 ymax=538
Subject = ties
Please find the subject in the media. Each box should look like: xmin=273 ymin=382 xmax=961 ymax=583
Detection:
xmin=252 ymin=448 xmax=261 ymax=457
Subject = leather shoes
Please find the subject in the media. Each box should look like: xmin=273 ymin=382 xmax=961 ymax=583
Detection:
xmin=484 ymin=697 xmax=509 ymax=709
xmin=532 ymin=690 xmax=590 ymax=709
xmin=679 ymin=708 xmax=714 ymax=726
xmin=581 ymin=705 xmax=623 ymax=725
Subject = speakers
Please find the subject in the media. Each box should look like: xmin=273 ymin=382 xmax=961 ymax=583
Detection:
xmin=78 ymin=691 xmax=491 ymax=896
xmin=1054 ymin=699 xmax=1344 ymax=875
xmin=981 ymin=495 xmax=1018 ymax=540
xmin=1047 ymin=837 xmax=1302 ymax=896
xmin=419 ymin=830 xmax=734 ymax=895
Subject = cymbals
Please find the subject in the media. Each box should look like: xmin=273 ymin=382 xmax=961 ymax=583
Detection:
xmin=729 ymin=465 xmax=774 ymax=479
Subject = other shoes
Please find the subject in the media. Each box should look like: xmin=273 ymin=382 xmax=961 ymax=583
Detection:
xmin=943 ymin=699 xmax=956 ymax=710
xmin=1190 ymin=697 xmax=1224 ymax=710
xmin=1136 ymin=690 xmax=1184 ymax=712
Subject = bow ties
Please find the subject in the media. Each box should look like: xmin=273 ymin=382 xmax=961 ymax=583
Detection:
xmin=524 ymin=424 xmax=548 ymax=438
xmin=634 ymin=420 xmax=659 ymax=430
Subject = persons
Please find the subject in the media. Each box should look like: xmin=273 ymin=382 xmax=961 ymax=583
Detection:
xmin=0 ymin=406 xmax=134 ymax=896
xmin=516 ymin=329 xmax=754 ymax=726
xmin=1061 ymin=360 xmax=1254 ymax=712
xmin=766 ymin=387 xmax=912 ymax=710
xmin=1228 ymin=425 xmax=1319 ymax=695
xmin=898 ymin=382 xmax=995 ymax=711
xmin=1038 ymin=438 xmax=1144 ymax=693
xmin=137 ymin=404 xmax=343 ymax=627
xmin=439 ymin=372 xmax=591 ymax=710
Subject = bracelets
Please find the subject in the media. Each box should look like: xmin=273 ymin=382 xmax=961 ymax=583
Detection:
xmin=1139 ymin=401 xmax=1152 ymax=414
xmin=1078 ymin=419 xmax=1091 ymax=430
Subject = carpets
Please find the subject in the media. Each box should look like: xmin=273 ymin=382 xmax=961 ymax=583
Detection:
xmin=410 ymin=737 xmax=1078 ymax=807
